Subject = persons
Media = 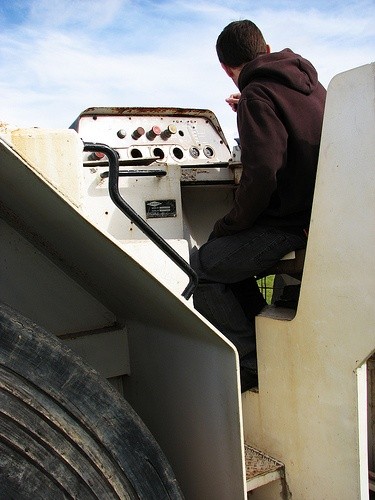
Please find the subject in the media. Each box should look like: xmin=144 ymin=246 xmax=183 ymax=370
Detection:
xmin=191 ymin=19 xmax=327 ymax=398
